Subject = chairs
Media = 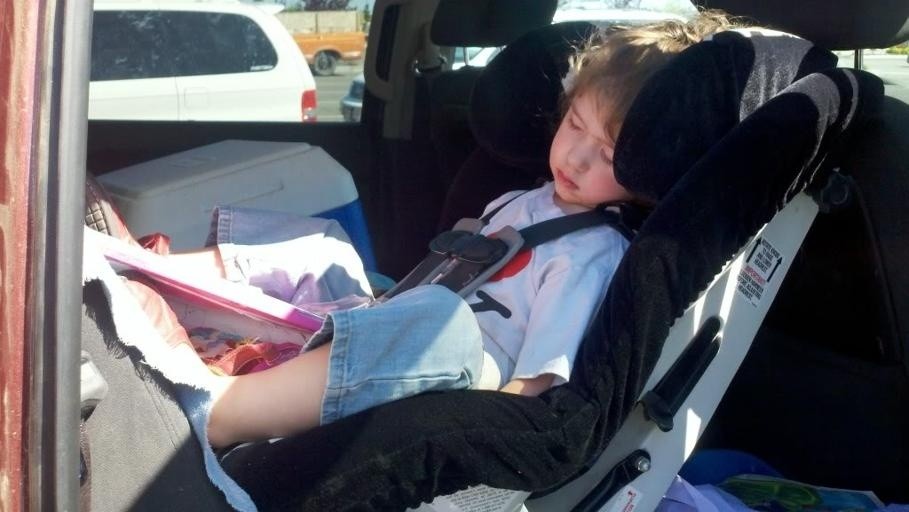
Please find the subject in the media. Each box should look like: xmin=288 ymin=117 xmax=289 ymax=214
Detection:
xmin=221 ymin=0 xmax=909 ymax=512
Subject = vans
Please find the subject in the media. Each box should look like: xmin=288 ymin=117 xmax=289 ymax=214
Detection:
xmin=87 ymin=4 xmax=365 ymax=122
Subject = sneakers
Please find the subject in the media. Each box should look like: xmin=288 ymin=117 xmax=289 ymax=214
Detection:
xmin=83 ymin=169 xmax=168 ymax=255
xmin=127 ymin=277 xmax=192 ymax=355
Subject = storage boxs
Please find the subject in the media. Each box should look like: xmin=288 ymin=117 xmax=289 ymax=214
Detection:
xmin=94 ymin=140 xmax=379 ymax=275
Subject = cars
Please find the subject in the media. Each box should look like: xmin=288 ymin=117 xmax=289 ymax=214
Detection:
xmin=340 ymin=10 xmax=688 ymax=122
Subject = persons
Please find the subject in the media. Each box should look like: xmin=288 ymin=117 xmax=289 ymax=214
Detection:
xmin=83 ymin=9 xmax=737 ymax=450
